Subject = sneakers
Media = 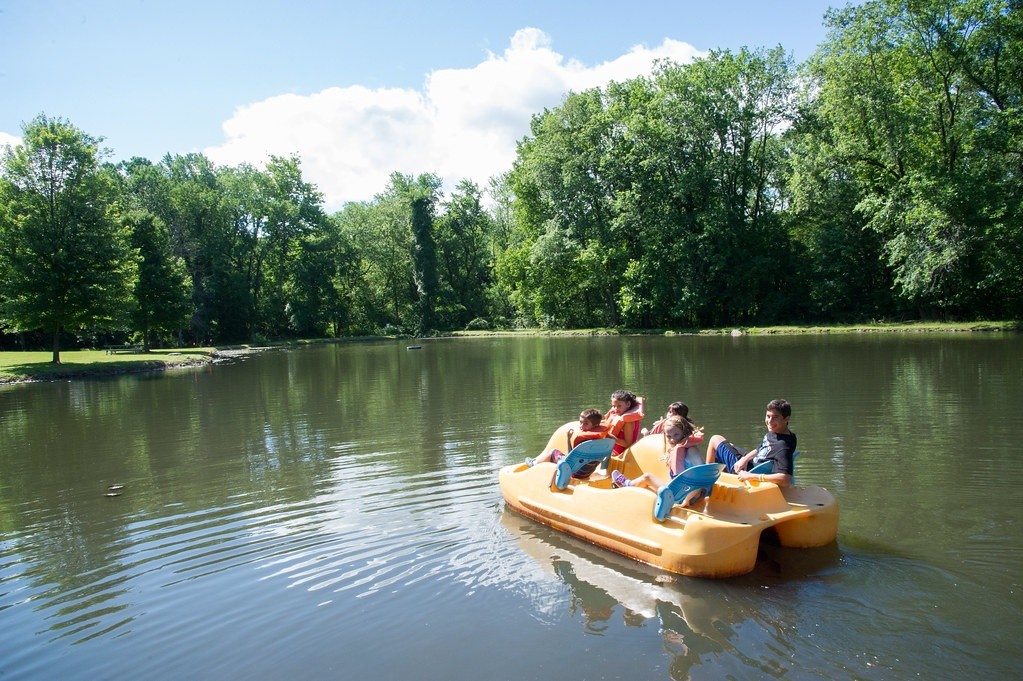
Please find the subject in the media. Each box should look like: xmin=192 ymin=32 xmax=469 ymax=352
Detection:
xmin=612 ymin=470 xmax=626 ymax=488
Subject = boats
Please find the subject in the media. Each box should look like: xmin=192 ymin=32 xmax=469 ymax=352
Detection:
xmin=500 ymin=506 xmax=766 ymax=636
xmin=498 ymin=421 xmax=840 ymax=583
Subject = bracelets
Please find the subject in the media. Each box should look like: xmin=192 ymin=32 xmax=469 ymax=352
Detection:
xmin=759 ymin=474 xmax=766 ymax=482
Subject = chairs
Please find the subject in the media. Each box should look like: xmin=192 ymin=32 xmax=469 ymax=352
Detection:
xmin=554 ymin=438 xmax=617 ymax=491
xmin=749 ymin=451 xmax=800 ymax=485
xmin=651 ymin=462 xmax=727 ymax=523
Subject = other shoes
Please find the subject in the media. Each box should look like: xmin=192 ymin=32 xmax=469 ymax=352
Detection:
xmin=525 ymin=456 xmax=535 ymax=468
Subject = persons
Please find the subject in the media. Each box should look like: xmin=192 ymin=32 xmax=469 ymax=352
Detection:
xmin=706 ymin=400 xmax=797 ymax=488
xmin=641 ymin=401 xmax=694 ymax=436
xmin=525 ymin=409 xmax=607 ymax=478
xmin=611 ymin=415 xmax=703 ymax=508
xmin=604 ymin=389 xmax=640 ymax=457
